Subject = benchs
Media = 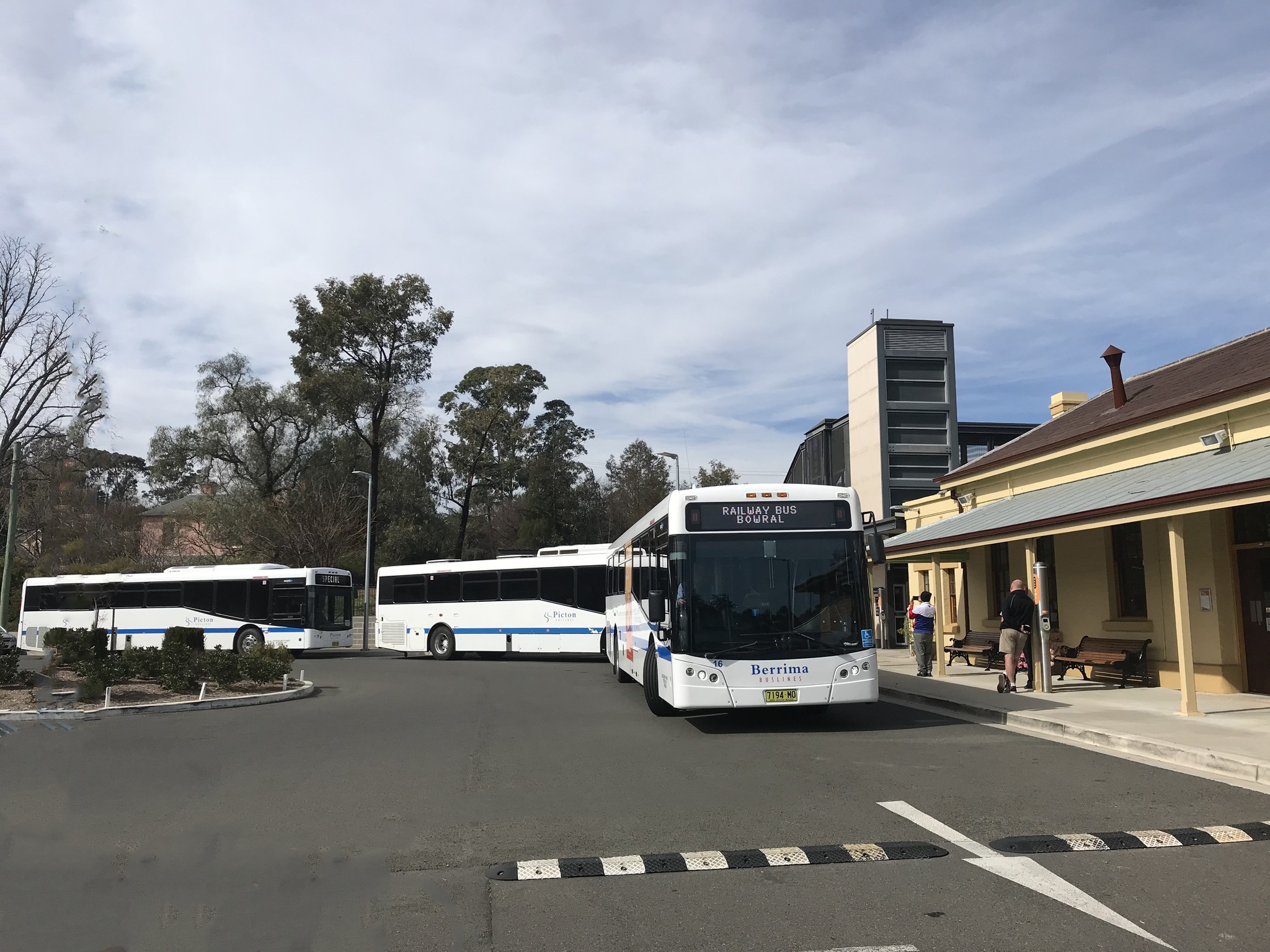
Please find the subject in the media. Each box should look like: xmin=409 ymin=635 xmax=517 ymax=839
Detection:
xmin=1052 ymin=636 xmax=1153 ymax=689
xmin=943 ymin=630 xmax=1006 ymax=671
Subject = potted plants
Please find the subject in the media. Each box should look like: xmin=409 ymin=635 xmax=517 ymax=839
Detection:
xmin=897 ymin=611 xmax=936 ymax=660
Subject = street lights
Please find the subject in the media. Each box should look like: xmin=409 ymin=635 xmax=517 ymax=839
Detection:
xmin=656 ymin=452 xmax=679 ymax=489
xmin=351 ymin=471 xmax=372 ymax=651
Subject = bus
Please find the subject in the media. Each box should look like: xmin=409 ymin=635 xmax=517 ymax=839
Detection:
xmin=17 ymin=564 xmax=359 ymax=660
xmin=374 ymin=543 xmax=643 ymax=661
xmin=605 ymin=483 xmax=886 ymax=719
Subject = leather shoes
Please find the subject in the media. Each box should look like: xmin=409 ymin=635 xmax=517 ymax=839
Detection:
xmin=925 ymin=672 xmax=932 ymax=677
xmin=1024 ymin=685 xmax=1032 ymax=689
xmin=917 ymin=671 xmax=925 ymax=676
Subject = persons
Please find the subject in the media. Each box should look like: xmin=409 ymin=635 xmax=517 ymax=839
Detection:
xmin=909 ymin=591 xmax=936 ymax=678
xmin=996 ymin=579 xmax=1036 ymax=693
xmin=999 ymin=584 xmax=1033 ymax=689
xmin=741 ymin=576 xmax=766 ymax=608
xmin=676 ymin=561 xmax=707 ymax=605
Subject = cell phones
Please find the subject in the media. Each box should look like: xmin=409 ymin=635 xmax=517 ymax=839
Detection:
xmin=913 ymin=596 xmax=918 ymax=600
xmin=1023 ymin=625 xmax=1032 ymax=633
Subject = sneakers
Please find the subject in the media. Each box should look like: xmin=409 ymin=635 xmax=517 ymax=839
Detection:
xmin=1004 ymin=684 xmax=1017 ymax=693
xmin=997 ymin=674 xmax=1006 ymax=693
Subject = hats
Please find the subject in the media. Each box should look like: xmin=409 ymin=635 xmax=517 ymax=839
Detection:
xmin=920 ymin=591 xmax=932 ymax=602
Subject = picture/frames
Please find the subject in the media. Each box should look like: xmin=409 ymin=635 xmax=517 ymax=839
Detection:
xmin=1198 ymin=588 xmax=1213 ymax=612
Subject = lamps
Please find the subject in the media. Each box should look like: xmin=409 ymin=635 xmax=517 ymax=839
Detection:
xmin=958 ymin=493 xmax=976 ymax=505
xmin=1198 ymin=429 xmax=1232 ymax=447
xmin=890 ymin=505 xmax=920 ymax=514
